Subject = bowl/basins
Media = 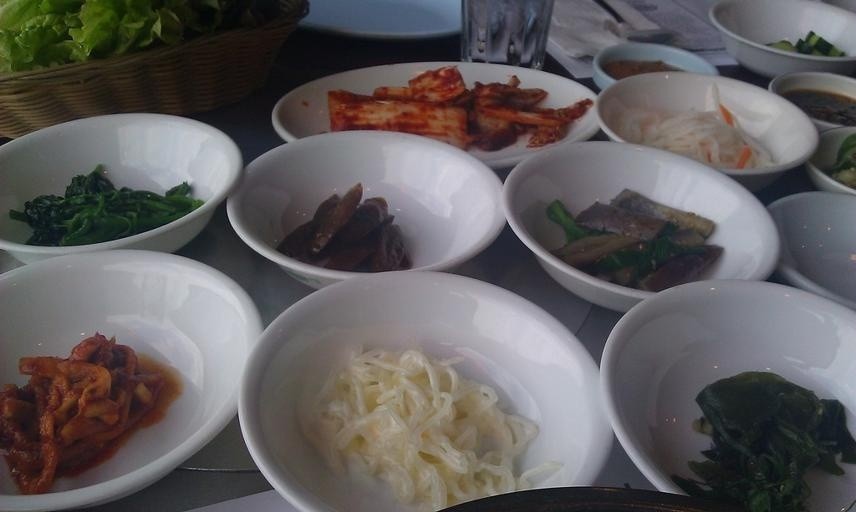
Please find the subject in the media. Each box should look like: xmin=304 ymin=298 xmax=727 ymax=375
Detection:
xmin=0 ymin=249 xmax=266 ymax=512
xmin=0 ymin=112 xmax=243 ymax=264
xmin=594 ymin=71 xmax=820 ymax=195
xmin=500 ymin=141 xmax=782 ymax=313
xmin=227 ymin=130 xmax=507 ymax=290
xmin=600 ymin=279 xmax=856 ymax=512
xmin=708 ymin=0 xmax=856 ymax=80
xmin=238 ymin=271 xmax=614 ymax=512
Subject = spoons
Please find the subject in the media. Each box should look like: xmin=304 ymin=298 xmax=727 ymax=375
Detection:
xmin=593 ymin=0 xmax=685 ymax=50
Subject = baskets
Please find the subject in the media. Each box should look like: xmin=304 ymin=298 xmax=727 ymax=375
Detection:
xmin=0 ymin=0 xmax=314 ymax=144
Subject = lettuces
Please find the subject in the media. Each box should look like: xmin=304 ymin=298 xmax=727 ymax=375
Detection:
xmin=0 ymin=0 xmax=301 ymax=71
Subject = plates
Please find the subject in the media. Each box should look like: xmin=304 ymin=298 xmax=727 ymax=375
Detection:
xmin=767 ymin=71 xmax=856 ymax=132
xmin=766 ymin=191 xmax=856 ymax=310
xmin=591 ymin=42 xmax=720 ymax=90
xmin=271 ymin=62 xmax=601 ymax=183
xmin=298 ymin=0 xmax=461 ymax=44
xmin=804 ymin=127 xmax=856 ymax=195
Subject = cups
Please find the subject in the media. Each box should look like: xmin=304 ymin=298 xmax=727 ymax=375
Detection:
xmin=460 ymin=0 xmax=555 ymax=71
